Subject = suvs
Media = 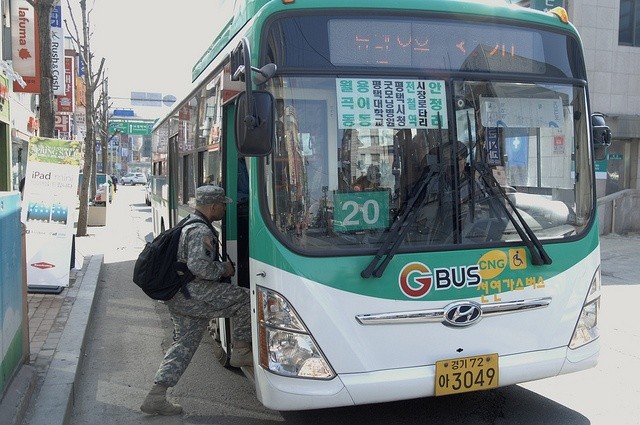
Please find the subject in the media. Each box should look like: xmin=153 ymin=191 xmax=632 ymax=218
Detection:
xmin=119 ymin=171 xmax=148 ymax=185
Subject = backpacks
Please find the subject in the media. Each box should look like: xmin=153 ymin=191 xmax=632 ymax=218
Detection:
xmin=133 ymin=214 xmax=219 ymax=301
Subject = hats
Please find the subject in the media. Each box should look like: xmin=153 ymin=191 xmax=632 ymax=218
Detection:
xmin=195 ymin=185 xmax=233 ymax=205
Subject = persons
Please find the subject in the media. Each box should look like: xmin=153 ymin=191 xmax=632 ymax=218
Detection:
xmin=443 ymin=139 xmax=489 ymax=205
xmin=139 ymin=185 xmax=254 ymax=417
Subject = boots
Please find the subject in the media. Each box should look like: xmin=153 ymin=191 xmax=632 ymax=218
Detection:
xmin=140 ymin=383 xmax=183 ymax=416
xmin=230 ymin=337 xmax=253 ymax=368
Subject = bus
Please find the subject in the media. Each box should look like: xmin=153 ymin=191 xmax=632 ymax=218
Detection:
xmin=149 ymin=0 xmax=613 ymax=412
